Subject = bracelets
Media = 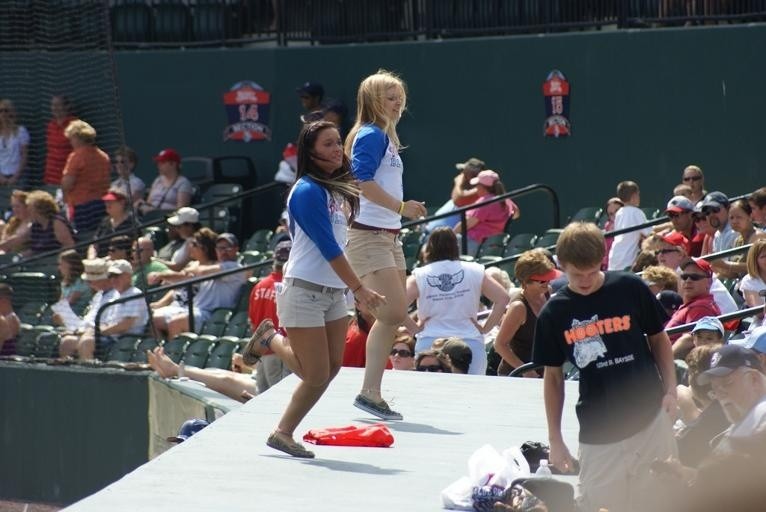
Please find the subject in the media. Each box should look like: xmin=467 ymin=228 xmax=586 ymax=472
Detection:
xmin=352 ymin=285 xmax=362 ymax=294
xmin=400 ymin=203 xmax=407 ymax=214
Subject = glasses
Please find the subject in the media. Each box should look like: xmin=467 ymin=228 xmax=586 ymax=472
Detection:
xmin=682 ymin=273 xmax=706 ymax=280
xmin=693 ymin=207 xmax=719 ymax=222
xmin=418 ymin=365 xmax=440 ymax=372
xmin=683 ymin=175 xmax=701 ymax=181
xmin=656 ymin=248 xmax=678 ymax=255
xmin=390 ymin=348 xmax=410 ymax=357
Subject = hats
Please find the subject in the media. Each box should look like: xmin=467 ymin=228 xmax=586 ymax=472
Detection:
xmin=275 ymin=242 xmax=292 ymax=264
xmin=653 ymin=232 xmax=691 ymax=253
xmin=217 ymin=233 xmax=238 ymax=247
xmin=155 ymin=149 xmax=178 ymax=162
xmin=690 ymin=316 xmax=766 ymax=386
xmin=655 ymin=290 xmax=683 ymax=310
xmin=665 ymin=191 xmax=727 ymax=212
xmin=531 ymin=269 xmax=562 ymax=282
xmin=101 ymin=191 xmax=125 ymax=201
xmin=167 ymin=418 xmax=209 ymax=443
xmin=167 ymin=207 xmax=200 ymax=226
xmin=457 ymin=158 xmax=499 ymax=187
xmin=82 ymin=259 xmax=133 ymax=282
xmin=681 ymin=256 xmax=713 ymax=276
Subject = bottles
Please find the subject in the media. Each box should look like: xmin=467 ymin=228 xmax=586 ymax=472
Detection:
xmin=536 ymin=457 xmax=554 ymax=479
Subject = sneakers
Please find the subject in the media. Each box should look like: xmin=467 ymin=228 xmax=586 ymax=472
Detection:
xmin=242 ymin=319 xmax=275 ymax=365
xmin=352 ymin=392 xmax=403 ymax=421
xmin=267 ymin=435 xmax=314 ymax=458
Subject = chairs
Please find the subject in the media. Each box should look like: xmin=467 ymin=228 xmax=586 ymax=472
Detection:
xmin=401 ymin=200 xmax=660 ymax=292
xmin=0 ymin=180 xmax=289 ymax=370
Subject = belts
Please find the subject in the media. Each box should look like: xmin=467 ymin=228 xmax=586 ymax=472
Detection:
xmin=287 ymin=276 xmax=341 ymax=293
xmin=348 ymin=221 xmax=401 ymax=235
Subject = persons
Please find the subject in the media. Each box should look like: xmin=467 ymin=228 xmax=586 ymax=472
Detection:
xmin=242 ymin=122 xmax=388 ymax=459
xmin=657 ymin=342 xmax=766 ymax=512
xmin=0 ymin=79 xmax=349 ymax=449
xmin=342 ymin=67 xmax=429 ymax=421
xmin=342 ymin=296 xmax=392 ymax=369
xmin=388 ymin=157 xmax=766 ymax=384
xmin=532 ymin=220 xmax=682 ymax=512
